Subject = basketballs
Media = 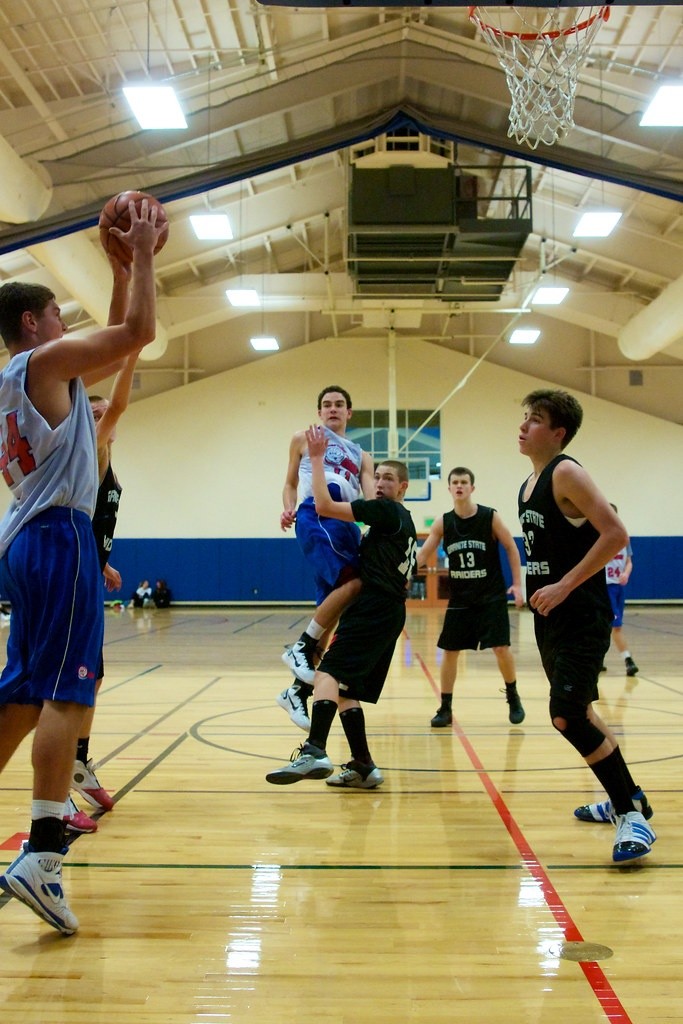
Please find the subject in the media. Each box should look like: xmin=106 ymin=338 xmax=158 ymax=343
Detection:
xmin=98 ymin=190 xmax=171 ymax=262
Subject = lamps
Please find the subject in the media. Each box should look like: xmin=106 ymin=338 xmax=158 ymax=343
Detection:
xmin=530 ymin=167 xmax=571 ymax=305
xmin=249 ymin=302 xmax=281 ymax=351
xmin=508 ymin=327 xmax=541 ymax=345
xmin=120 ymin=0 xmax=190 ymax=130
xmin=226 ymin=179 xmax=261 ymax=308
xmin=572 ymin=46 xmax=623 ymax=239
xmin=190 ymin=51 xmax=232 ymax=242
xmin=638 ymin=81 xmax=683 ymax=128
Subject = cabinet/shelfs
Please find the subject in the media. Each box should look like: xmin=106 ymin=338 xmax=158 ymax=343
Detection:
xmin=404 ymin=533 xmax=449 ymax=608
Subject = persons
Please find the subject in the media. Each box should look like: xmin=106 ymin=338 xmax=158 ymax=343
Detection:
xmin=148 ymin=580 xmax=170 ymax=610
xmin=603 ymin=501 xmax=640 ymax=675
xmin=278 ymin=386 xmax=376 ymax=732
xmin=516 ymin=389 xmax=659 ymax=862
xmin=126 ymin=579 xmax=152 ymax=608
xmin=265 ymin=422 xmax=418 ymax=787
xmin=60 ymin=347 xmax=141 ymax=834
xmin=414 ymin=467 xmax=524 ymax=727
xmin=1 ymin=199 xmax=165 ymax=938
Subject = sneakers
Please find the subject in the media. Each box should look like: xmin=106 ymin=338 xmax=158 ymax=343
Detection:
xmin=63 ymin=796 xmax=97 ymax=833
xmin=499 ymin=686 xmax=524 ymax=724
xmin=573 ymin=786 xmax=652 ymax=821
xmin=612 ymin=807 xmax=657 ymax=863
xmin=325 ymin=759 xmax=383 ymax=787
xmin=266 ymin=742 xmax=332 ymax=784
xmin=431 ymin=706 xmax=452 ymax=726
xmin=281 ymin=639 xmax=318 ymax=688
xmin=276 ymin=686 xmax=311 ymax=732
xmin=69 ymin=759 xmax=114 ymax=813
xmin=0 ymin=845 xmax=79 ymax=938
xmin=626 ymin=659 xmax=637 ymax=675
